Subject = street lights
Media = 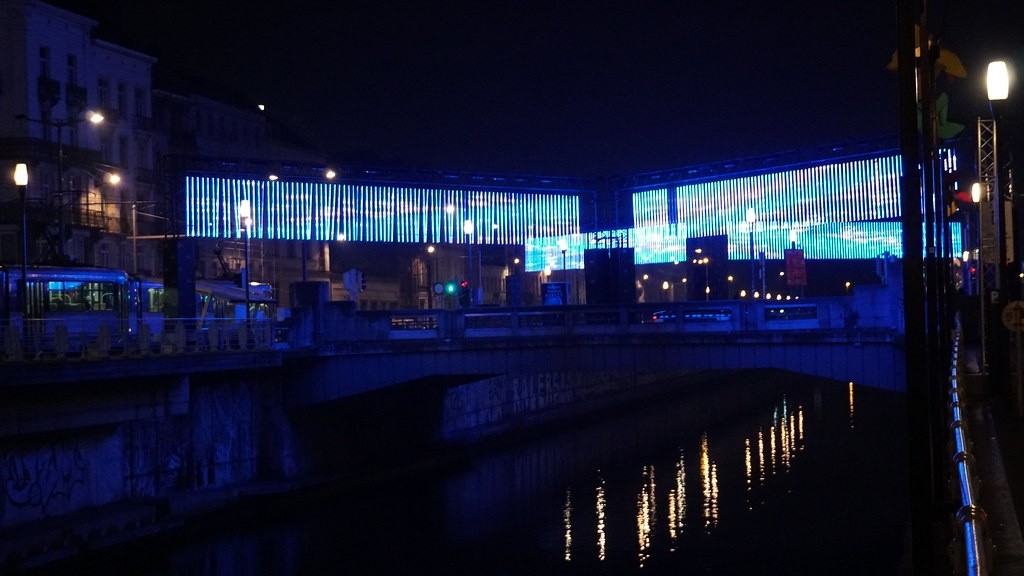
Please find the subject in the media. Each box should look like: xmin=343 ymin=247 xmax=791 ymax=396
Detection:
xmin=240 ymin=200 xmax=256 ymax=348
xmin=561 ymin=240 xmax=568 ymax=304
xmin=16 ymin=109 xmax=102 ymax=267
xmin=464 ymin=220 xmax=474 ymax=306
xmin=13 ymin=163 xmax=29 ymax=362
xmin=987 ymin=59 xmax=1011 ymax=393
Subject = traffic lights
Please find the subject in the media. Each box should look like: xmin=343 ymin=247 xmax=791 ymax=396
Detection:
xmin=447 ymin=281 xmax=456 ymax=293
xmin=460 ymin=280 xmax=470 ymax=307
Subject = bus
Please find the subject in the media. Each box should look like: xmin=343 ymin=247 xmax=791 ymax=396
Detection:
xmin=0 ymin=265 xmax=141 ymax=355
